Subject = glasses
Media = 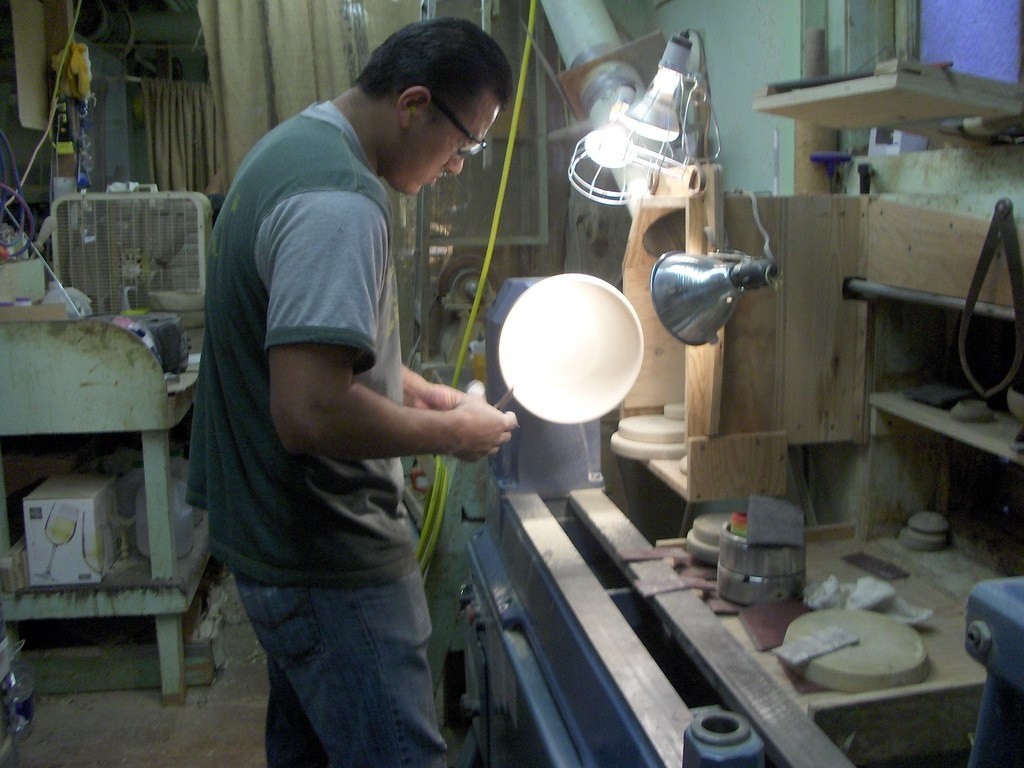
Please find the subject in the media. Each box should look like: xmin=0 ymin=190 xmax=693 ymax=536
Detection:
xmin=434 ymin=96 xmax=488 ymax=157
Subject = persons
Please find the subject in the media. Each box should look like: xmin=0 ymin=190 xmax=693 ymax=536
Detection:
xmin=187 ymin=18 xmax=517 ymax=768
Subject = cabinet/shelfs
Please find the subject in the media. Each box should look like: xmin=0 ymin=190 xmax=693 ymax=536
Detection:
xmin=0 ymin=317 xmax=213 ymax=710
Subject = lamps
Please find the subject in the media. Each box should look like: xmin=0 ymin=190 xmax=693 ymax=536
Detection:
xmin=562 ymin=28 xmax=786 ymax=345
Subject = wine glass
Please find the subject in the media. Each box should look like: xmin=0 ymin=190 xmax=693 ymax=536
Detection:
xmin=33 ymin=501 xmax=80 ymax=581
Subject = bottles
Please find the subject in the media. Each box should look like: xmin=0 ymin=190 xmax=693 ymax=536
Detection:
xmin=135 ymin=470 xmax=194 ymax=558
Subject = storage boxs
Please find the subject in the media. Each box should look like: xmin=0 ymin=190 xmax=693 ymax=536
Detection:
xmin=20 ymin=610 xmax=224 ymax=698
xmin=19 ymin=471 xmax=121 ymax=588
